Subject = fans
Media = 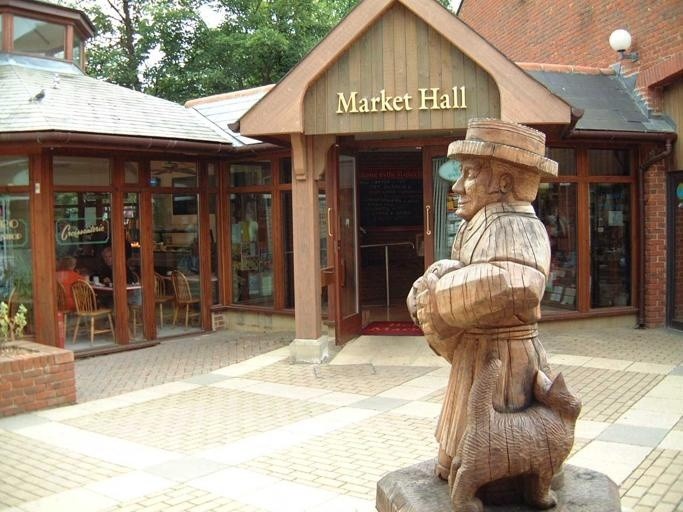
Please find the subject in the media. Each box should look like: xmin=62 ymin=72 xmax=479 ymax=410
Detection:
xmin=152 ymin=160 xmax=196 ymax=178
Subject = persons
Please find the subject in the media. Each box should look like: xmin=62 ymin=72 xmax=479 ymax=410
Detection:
xmin=56 ymin=218 xmax=137 ymax=311
xmin=406 ymin=116 xmax=559 ymax=488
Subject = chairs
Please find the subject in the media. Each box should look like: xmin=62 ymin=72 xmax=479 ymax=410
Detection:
xmin=3 ymin=259 xmax=205 ymax=346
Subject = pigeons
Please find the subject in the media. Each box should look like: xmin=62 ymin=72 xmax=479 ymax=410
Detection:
xmin=51 ymin=73 xmax=60 ymax=89
xmin=27 ymin=88 xmax=46 ymax=103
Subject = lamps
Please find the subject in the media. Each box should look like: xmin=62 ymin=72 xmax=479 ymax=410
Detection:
xmin=607 ymin=28 xmax=639 ymax=64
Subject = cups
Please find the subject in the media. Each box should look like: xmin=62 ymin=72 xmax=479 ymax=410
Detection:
xmin=93 ymin=276 xmax=100 ymax=285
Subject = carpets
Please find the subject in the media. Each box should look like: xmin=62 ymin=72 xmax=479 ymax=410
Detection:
xmin=358 ymin=318 xmax=427 ymax=338
